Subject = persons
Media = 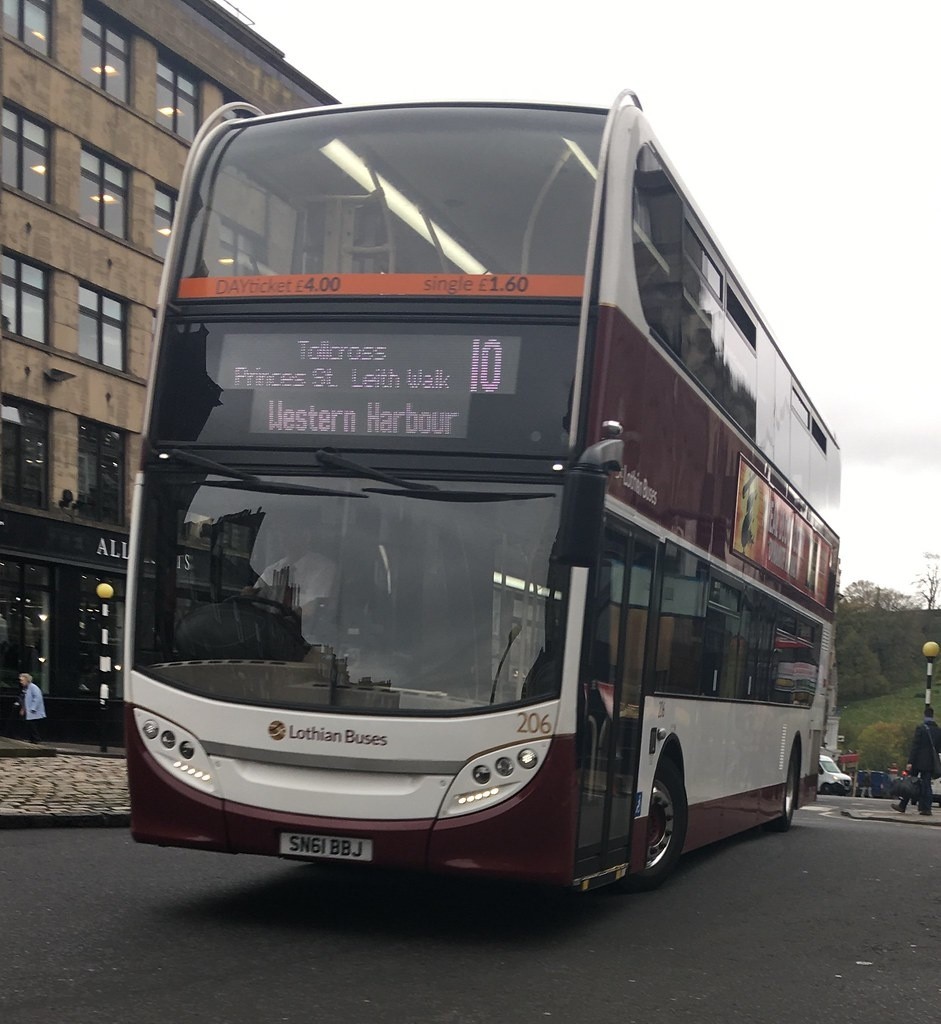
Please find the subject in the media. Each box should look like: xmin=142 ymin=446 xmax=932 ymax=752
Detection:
xmin=860 ymin=768 xmax=873 ymax=798
xmin=17 ymin=673 xmax=46 ymax=744
xmin=890 ymin=708 xmax=941 ymax=816
xmin=238 ymin=509 xmax=340 ymax=653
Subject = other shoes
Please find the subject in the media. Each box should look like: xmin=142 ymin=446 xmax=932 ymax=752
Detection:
xmin=891 ymin=804 xmax=903 ymax=812
xmin=920 ymin=812 xmax=930 ymax=815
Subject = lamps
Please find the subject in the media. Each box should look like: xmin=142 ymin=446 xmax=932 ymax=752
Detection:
xmin=59 ymin=489 xmax=95 ymax=511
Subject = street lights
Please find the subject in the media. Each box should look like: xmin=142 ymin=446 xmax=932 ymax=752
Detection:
xmin=922 ymin=642 xmax=939 ymax=719
xmin=97 ymin=582 xmax=116 ymax=754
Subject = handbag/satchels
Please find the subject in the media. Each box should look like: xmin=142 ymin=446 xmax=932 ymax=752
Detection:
xmin=891 ymin=770 xmax=921 ymax=800
xmin=931 ymin=752 xmax=941 ymax=779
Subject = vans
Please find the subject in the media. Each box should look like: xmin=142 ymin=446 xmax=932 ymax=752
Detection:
xmin=819 ymin=755 xmax=857 ymax=796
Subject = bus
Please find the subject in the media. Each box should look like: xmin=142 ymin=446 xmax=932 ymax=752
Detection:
xmin=116 ymin=95 xmax=839 ymax=892
